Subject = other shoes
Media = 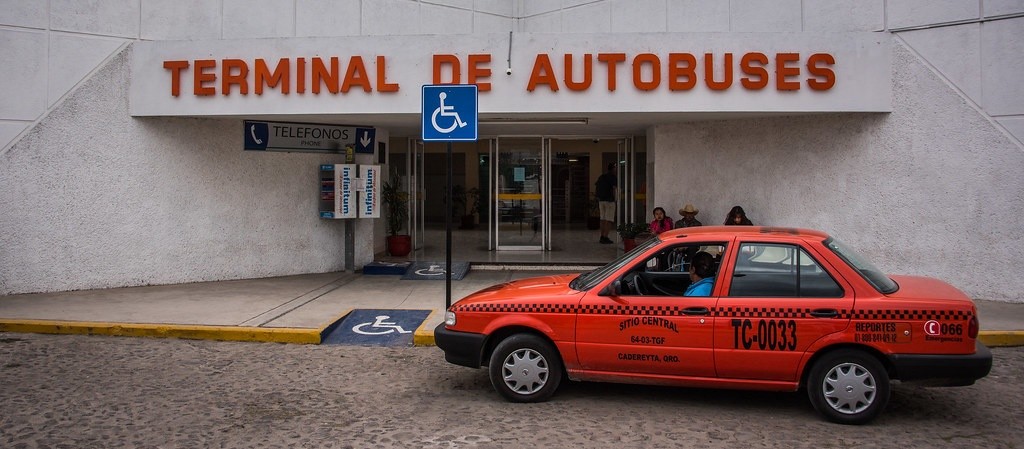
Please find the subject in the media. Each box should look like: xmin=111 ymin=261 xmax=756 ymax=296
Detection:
xmin=599 ymin=235 xmax=614 ymax=245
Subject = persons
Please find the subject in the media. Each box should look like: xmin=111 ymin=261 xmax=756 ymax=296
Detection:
xmin=649 ymin=204 xmax=753 ymax=272
xmin=683 ymin=251 xmax=716 ymax=296
xmin=594 ymin=161 xmax=617 ymax=244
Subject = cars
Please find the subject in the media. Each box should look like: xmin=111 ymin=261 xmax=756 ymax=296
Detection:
xmin=434 ymin=226 xmax=995 ymax=426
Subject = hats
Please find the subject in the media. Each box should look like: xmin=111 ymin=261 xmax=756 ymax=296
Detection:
xmin=679 ymin=204 xmax=699 ymax=217
